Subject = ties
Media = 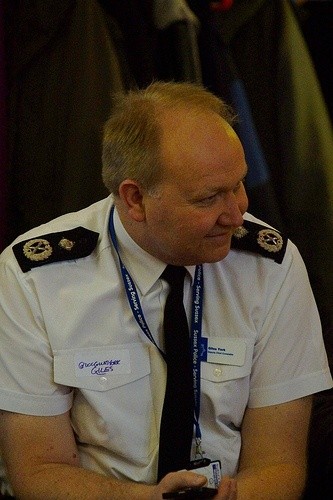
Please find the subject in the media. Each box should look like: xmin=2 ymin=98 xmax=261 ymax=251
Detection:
xmin=158 ymin=264 xmax=196 ymax=500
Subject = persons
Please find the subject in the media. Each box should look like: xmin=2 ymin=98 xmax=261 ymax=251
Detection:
xmin=0 ymin=82 xmax=333 ymax=500
